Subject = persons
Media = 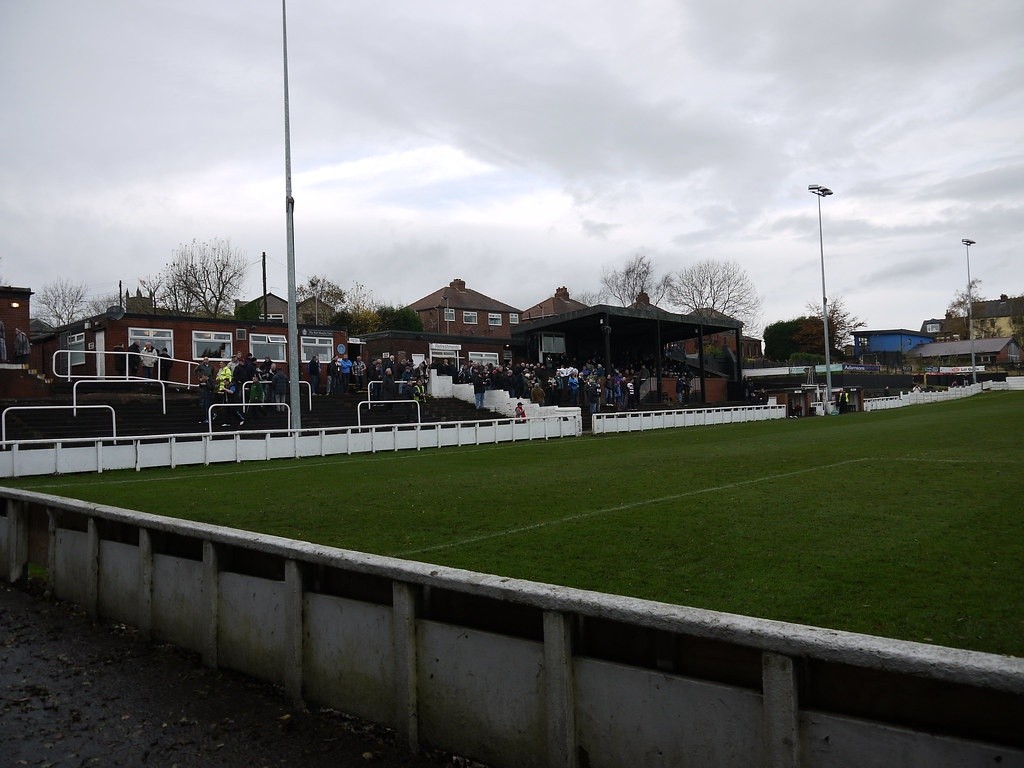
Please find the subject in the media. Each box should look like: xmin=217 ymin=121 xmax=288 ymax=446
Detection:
xmin=0 ymin=320 xmax=31 ymax=364
xmin=112 ymin=340 xmax=969 ymax=424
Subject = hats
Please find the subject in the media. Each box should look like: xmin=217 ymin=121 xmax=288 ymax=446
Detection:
xmin=479 ymin=370 xmax=484 ymax=374
xmin=162 ymin=348 xmax=167 ymax=353
xmin=517 ymin=402 xmax=524 ymax=405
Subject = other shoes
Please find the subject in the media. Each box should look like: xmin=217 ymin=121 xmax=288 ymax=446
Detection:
xmin=221 ymin=423 xmax=231 ymax=427
xmin=212 ymin=412 xmax=217 ymax=421
xmin=312 ymin=392 xmax=318 ymax=396
xmin=239 ymin=421 xmax=245 ymax=425
xmin=275 ymin=411 xmax=284 ymax=415
xmin=198 ymin=420 xmax=209 ymax=424
xmin=326 ymin=392 xmax=330 ymax=396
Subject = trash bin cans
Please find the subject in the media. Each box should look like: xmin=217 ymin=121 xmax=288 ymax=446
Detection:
xmin=826 ymin=401 xmax=837 ymax=414
xmin=843 ymin=386 xmax=862 ymax=411
xmin=810 ymin=402 xmax=824 ymax=416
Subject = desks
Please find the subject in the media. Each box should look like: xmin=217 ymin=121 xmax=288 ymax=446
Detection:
xmin=847 ymin=405 xmax=856 ymax=412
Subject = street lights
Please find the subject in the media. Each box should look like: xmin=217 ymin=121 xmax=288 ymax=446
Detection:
xmin=808 ymin=185 xmax=833 ymax=402
xmin=961 ymin=238 xmax=976 ymax=382
